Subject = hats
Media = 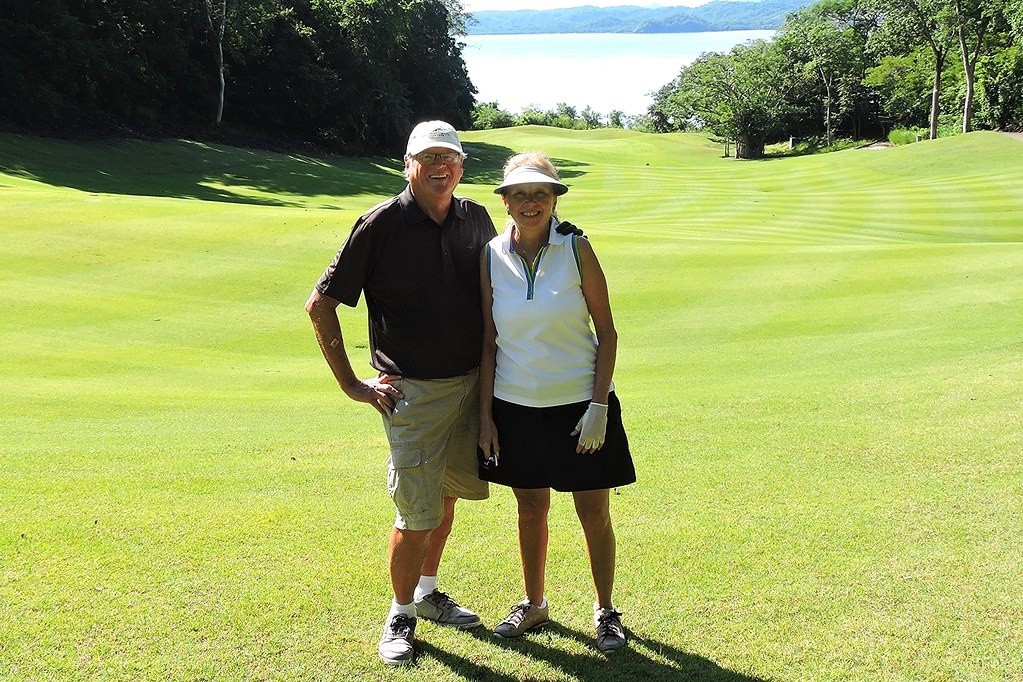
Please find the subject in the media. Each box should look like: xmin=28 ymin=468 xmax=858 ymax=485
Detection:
xmin=492 ymin=165 xmax=570 ymax=197
xmin=405 ymin=119 xmax=464 ymax=157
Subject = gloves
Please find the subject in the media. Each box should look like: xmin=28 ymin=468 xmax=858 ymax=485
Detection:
xmin=555 ymin=220 xmax=590 ymax=240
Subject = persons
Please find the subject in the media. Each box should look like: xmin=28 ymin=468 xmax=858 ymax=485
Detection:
xmin=478 ymin=152 xmax=636 ymax=650
xmin=306 ymin=120 xmax=589 ymax=666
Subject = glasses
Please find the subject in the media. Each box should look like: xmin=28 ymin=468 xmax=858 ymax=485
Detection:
xmin=416 ymin=151 xmax=462 ymax=166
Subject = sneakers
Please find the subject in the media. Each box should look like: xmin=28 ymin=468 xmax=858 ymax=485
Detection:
xmin=377 ymin=604 xmax=418 ymax=666
xmin=594 ymin=598 xmax=627 ymax=657
xmin=415 ymin=587 xmax=482 ymax=629
xmin=494 ymin=597 xmax=550 ymax=641
